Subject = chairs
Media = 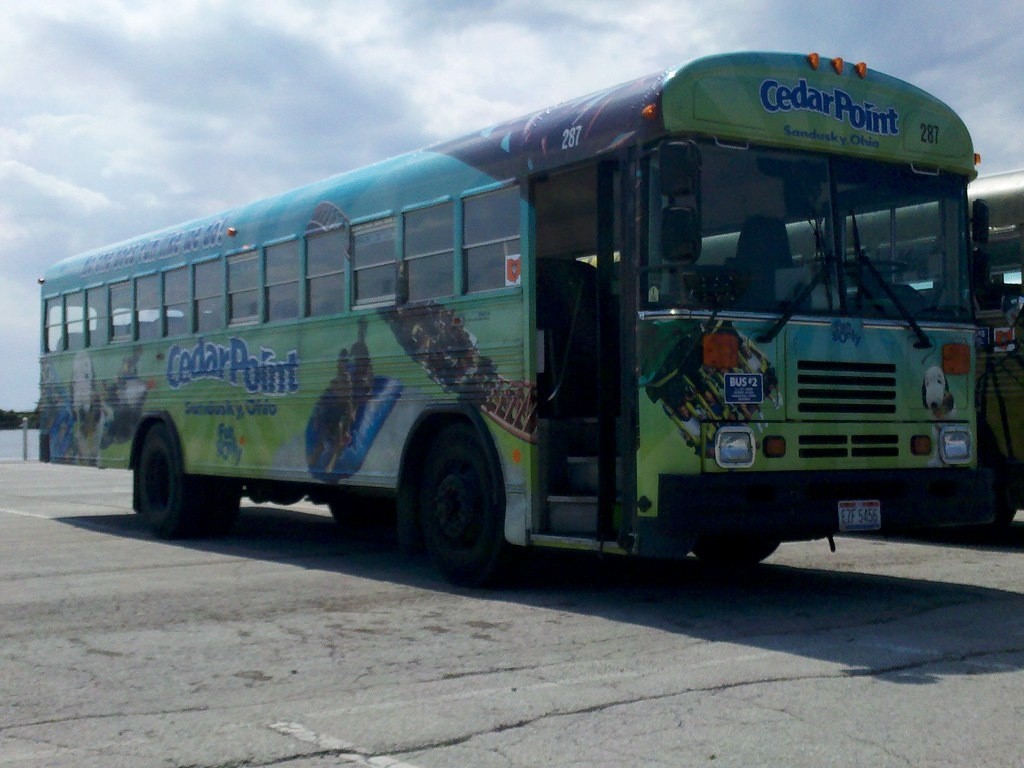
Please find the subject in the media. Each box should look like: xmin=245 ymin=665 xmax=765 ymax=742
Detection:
xmin=56 ymin=258 xmax=600 ymax=367
xmin=736 ymin=217 xmax=794 ymax=314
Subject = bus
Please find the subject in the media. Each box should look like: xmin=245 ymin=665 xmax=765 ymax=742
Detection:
xmin=37 ymin=50 xmax=980 ymax=597
xmin=563 ymin=168 xmax=1024 ymax=550
